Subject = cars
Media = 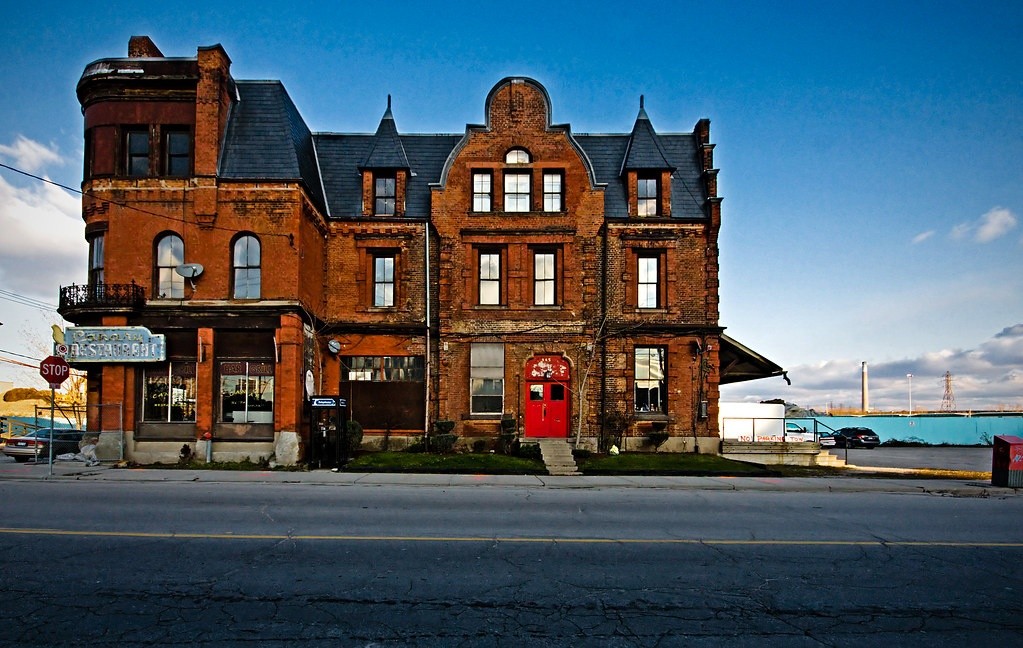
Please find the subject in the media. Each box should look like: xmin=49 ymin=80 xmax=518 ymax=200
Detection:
xmin=811 ymin=432 xmax=837 ymax=448
xmin=3 ymin=429 xmax=87 ymax=463
xmin=831 ymin=427 xmax=880 ymax=450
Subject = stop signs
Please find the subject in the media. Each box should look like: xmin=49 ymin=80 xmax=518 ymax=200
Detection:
xmin=39 ymin=355 xmax=71 ymax=385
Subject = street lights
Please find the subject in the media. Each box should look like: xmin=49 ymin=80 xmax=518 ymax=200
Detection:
xmin=906 ymin=373 xmax=913 ymax=414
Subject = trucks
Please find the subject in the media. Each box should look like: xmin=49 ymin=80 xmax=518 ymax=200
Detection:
xmin=719 ymin=402 xmax=822 ymax=443
